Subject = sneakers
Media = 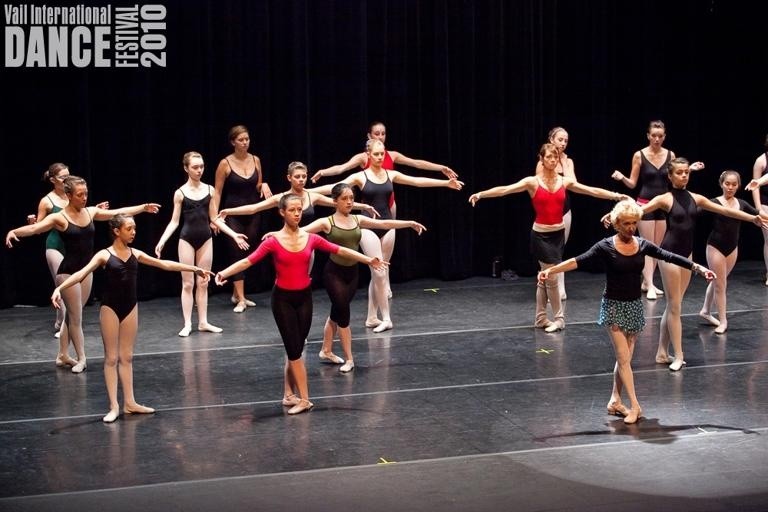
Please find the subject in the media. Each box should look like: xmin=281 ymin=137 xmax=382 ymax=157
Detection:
xmin=54 ymin=291 xmax=393 ymax=423
xmin=534 ymin=282 xmax=728 ymax=425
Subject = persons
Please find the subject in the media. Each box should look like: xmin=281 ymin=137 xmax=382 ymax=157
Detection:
xmin=5 ymin=176 xmax=162 ymax=374
xmin=468 ymin=120 xmax=767 ymax=425
xmin=27 ymin=162 xmax=110 ymax=340
xmin=51 ymin=211 xmax=217 ymax=424
xmin=154 ymin=122 xmax=464 ymax=416
